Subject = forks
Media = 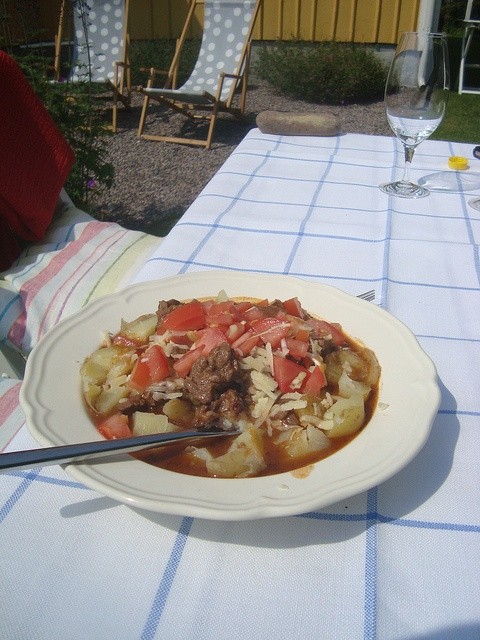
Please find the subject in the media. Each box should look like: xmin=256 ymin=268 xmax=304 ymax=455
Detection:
xmin=352 ymin=289 xmax=376 ymax=302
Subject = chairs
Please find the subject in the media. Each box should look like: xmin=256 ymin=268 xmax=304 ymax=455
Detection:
xmin=0 ymin=377 xmax=28 ymax=455
xmin=35 ymin=0 xmax=132 ymax=134
xmin=459 ymin=0 xmax=480 ymax=95
xmin=137 ymin=0 xmax=259 ymax=149
xmin=1 ymin=52 xmax=165 ymax=380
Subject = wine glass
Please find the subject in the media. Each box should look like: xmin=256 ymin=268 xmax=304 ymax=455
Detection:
xmin=377 ymin=31 xmax=451 ymax=202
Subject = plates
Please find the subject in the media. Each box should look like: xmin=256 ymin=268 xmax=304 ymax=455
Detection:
xmin=19 ymin=271 xmax=440 ymax=523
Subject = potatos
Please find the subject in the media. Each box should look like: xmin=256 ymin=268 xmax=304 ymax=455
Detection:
xmin=81 ymin=290 xmax=381 ymax=479
